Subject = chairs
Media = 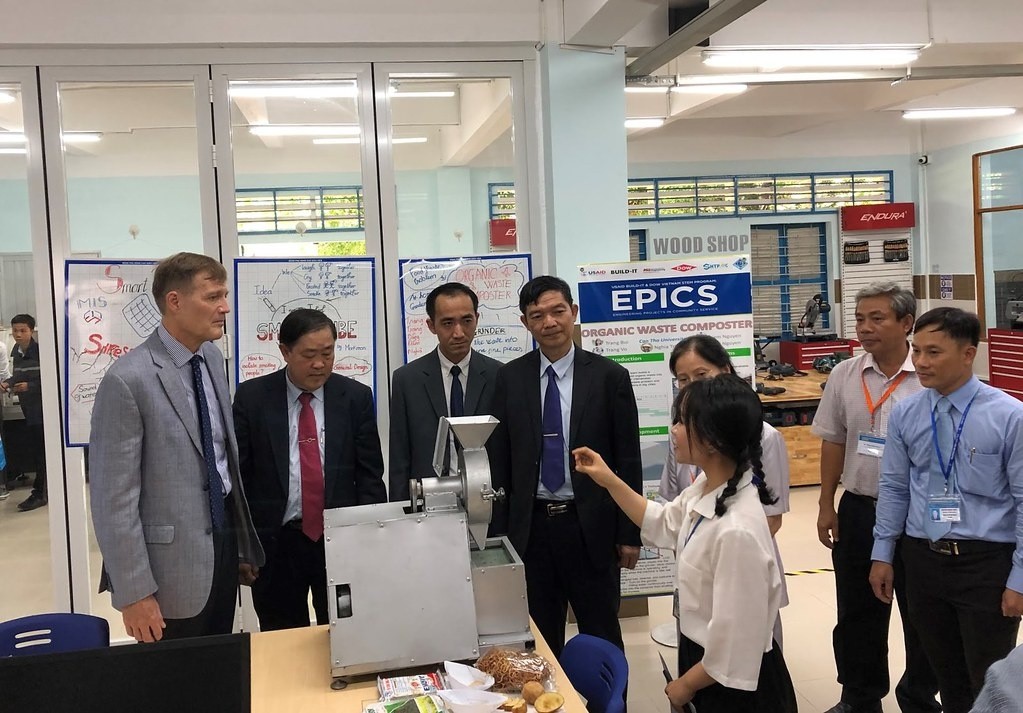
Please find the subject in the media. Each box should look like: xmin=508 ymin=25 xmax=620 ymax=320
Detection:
xmin=560 ymin=633 xmax=629 ymax=713
xmin=0 ymin=613 xmax=110 ymax=659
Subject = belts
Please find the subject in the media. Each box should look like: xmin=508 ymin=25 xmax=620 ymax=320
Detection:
xmin=534 ymin=501 xmax=575 ymax=516
xmin=907 ymin=536 xmax=1010 ymax=555
xmin=866 ymin=497 xmax=878 ymax=507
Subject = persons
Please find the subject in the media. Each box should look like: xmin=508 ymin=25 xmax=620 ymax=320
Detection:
xmin=969 ymin=643 xmax=1023 ymax=713
xmin=89 ymin=252 xmax=264 ymax=643
xmin=809 ymin=281 xmax=926 ymax=713
xmin=508 ymin=275 xmax=644 ymax=713
xmin=868 ymin=306 xmax=1023 ymax=713
xmin=234 ymin=308 xmax=388 ymax=631
xmin=659 ymin=334 xmax=791 ymax=654
xmin=388 ymin=281 xmax=536 ymax=557
xmin=0 ymin=314 xmax=48 ymax=511
xmin=932 ymin=511 xmax=940 ymax=520
xmin=572 ymin=373 xmax=798 ymax=713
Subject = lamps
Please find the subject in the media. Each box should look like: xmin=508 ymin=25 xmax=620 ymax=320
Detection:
xmin=0 ymin=42 xmax=1023 ymax=158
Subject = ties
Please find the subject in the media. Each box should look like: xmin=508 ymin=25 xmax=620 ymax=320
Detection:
xmin=298 ymin=392 xmax=324 ymax=543
xmin=190 ymin=357 xmax=227 ymax=536
xmin=448 ymin=364 xmax=463 ymax=456
xmin=540 ymin=365 xmax=565 ymax=493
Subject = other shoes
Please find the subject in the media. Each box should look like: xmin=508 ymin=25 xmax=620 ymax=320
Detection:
xmin=5 ymin=471 xmax=34 ymax=490
xmin=824 ymin=701 xmax=884 ymax=713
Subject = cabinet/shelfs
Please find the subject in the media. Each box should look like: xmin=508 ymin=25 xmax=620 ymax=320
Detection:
xmin=988 ymin=328 xmax=1023 ymax=403
xmin=780 ymin=339 xmax=861 ymax=370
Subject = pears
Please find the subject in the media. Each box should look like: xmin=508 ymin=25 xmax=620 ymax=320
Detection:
xmin=520 ymin=680 xmax=546 ymax=704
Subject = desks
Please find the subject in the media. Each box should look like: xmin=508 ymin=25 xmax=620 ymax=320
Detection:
xmin=756 ymin=369 xmax=831 ymax=486
xmin=250 ymin=614 xmax=589 ymax=713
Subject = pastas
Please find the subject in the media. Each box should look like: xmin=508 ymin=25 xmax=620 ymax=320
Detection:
xmin=478 ymin=650 xmax=551 ymax=692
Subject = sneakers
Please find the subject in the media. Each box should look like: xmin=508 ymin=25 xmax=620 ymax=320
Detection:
xmin=18 ymin=490 xmax=47 ymax=511
